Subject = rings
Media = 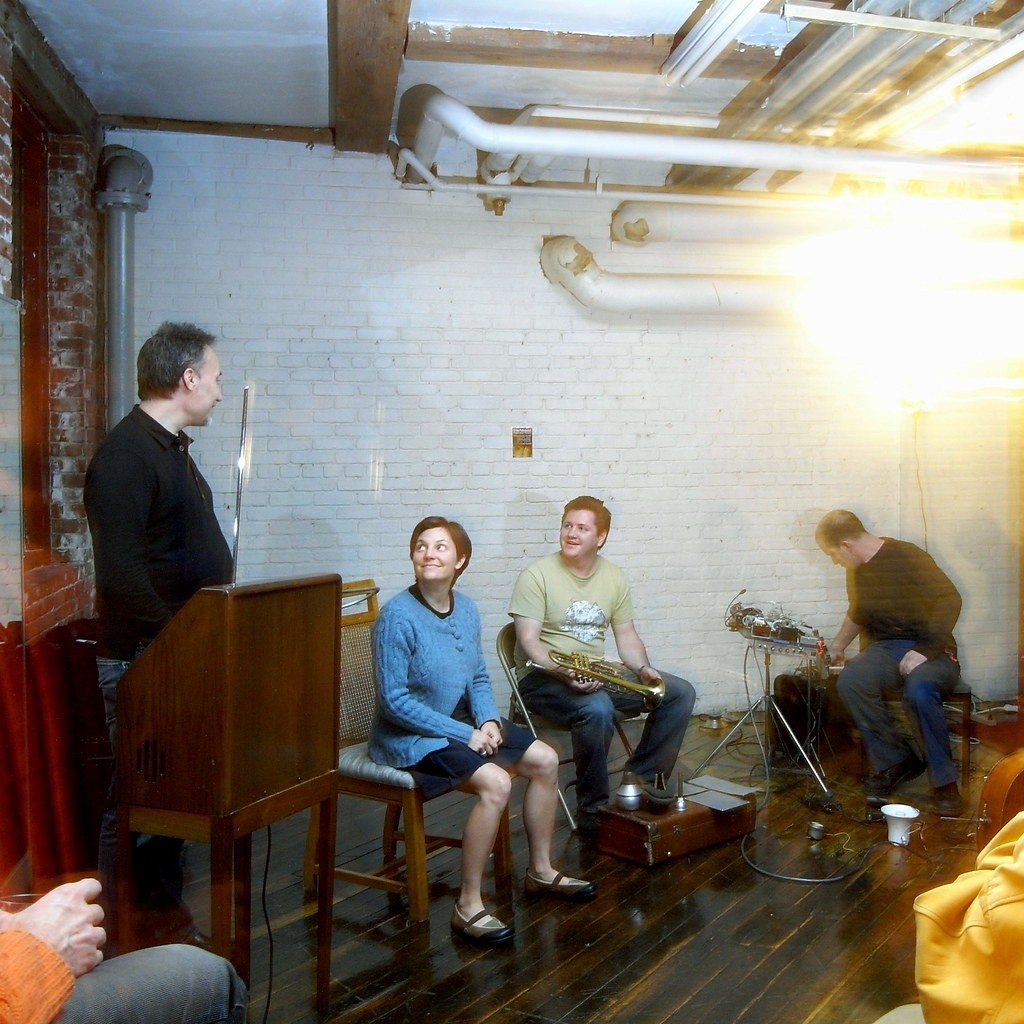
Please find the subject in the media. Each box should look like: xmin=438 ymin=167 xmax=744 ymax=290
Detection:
xmin=481 ymin=750 xmax=486 ymax=756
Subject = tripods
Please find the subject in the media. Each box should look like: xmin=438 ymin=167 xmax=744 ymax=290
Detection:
xmin=683 ymin=649 xmax=835 ymax=800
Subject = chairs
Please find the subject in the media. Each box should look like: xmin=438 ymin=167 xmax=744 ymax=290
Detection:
xmin=497 ymin=622 xmax=635 ymax=833
xmin=302 ymin=580 xmax=518 ymax=926
xmin=858 ymin=633 xmax=973 ymax=782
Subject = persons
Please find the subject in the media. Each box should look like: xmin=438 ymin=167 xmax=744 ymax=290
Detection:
xmin=368 ymin=516 xmax=597 ymax=945
xmin=0 ymin=878 xmax=249 ymax=1024
xmin=507 ymin=496 xmax=696 ymax=817
xmin=815 ymin=510 xmax=967 ymax=815
xmin=82 ymin=321 xmax=232 ymax=953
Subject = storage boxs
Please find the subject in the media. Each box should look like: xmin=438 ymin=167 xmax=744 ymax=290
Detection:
xmin=596 ymin=794 xmax=755 ymax=863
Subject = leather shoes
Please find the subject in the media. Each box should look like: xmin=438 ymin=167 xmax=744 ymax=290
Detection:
xmin=931 ymin=781 xmax=965 ymax=816
xmin=863 ymin=753 xmax=926 ymax=797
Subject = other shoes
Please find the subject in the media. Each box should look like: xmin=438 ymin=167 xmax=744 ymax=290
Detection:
xmin=189 ymin=932 xmax=238 ymax=967
xmin=523 ymin=868 xmax=596 ymax=900
xmin=641 ymin=781 xmax=676 ymax=805
xmin=449 ymin=903 xmax=515 ymax=943
xmin=572 ymin=803 xmax=609 ymax=837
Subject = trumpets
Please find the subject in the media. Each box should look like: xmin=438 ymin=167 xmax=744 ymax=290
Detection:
xmin=547 ymin=648 xmax=666 ymax=710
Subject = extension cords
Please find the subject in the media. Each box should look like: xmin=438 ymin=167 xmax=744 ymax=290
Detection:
xmin=1004 ymin=703 xmax=1018 ymax=712
xmin=968 ymin=711 xmax=996 ymax=726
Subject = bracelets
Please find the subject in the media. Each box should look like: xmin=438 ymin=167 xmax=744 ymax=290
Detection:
xmin=639 ymin=665 xmax=646 ymax=673
